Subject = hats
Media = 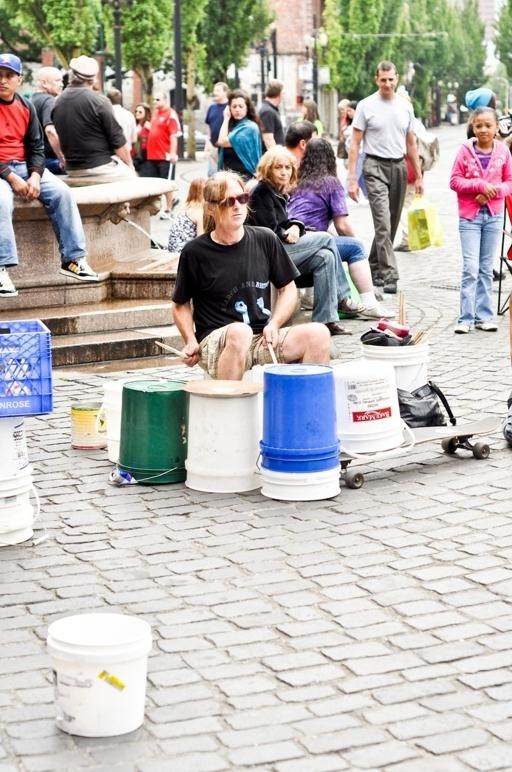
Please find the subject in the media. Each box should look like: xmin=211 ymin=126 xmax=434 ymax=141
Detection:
xmin=1 ymin=54 xmax=22 ymax=77
xmin=466 ymin=88 xmax=492 ymax=110
xmin=70 ymin=55 xmax=100 ymax=81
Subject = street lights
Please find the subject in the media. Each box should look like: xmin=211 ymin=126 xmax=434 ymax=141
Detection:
xmin=256 ymin=41 xmax=267 ymax=98
xmin=305 ymin=26 xmax=329 ymax=101
xmin=310 ymin=14 xmax=328 ymax=122
xmin=111 ymin=0 xmax=123 ymax=104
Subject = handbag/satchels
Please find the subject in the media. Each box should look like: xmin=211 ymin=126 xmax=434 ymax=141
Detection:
xmin=393 ymin=191 xmax=447 ymax=252
xmin=398 ymin=383 xmax=460 ymax=433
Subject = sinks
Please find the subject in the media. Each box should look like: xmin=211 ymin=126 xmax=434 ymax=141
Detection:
xmin=0 ymin=416 xmax=41 ymax=534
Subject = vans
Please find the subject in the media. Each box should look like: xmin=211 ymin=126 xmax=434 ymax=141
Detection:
xmin=104 ymin=75 xmax=216 ymax=152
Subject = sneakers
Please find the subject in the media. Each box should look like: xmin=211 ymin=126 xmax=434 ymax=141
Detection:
xmin=383 ymin=280 xmax=399 ymax=294
xmin=1 ymin=266 xmax=20 ymax=298
xmin=361 ymin=302 xmax=399 ymax=319
xmin=372 ymin=270 xmax=386 ymax=286
xmin=476 ymin=323 xmax=499 ymax=332
xmin=455 ymin=322 xmax=475 ymax=335
xmin=60 ymin=256 xmax=100 ymax=281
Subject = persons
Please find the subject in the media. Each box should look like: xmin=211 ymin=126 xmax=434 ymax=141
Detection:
xmin=131 ymin=102 xmax=152 ymax=171
xmin=0 ymin=53 xmax=108 ymax=300
xmin=464 ymin=86 xmax=512 ymax=147
xmin=171 ymin=166 xmax=333 ymax=382
xmin=166 ymin=176 xmax=206 ymax=253
xmin=243 ymin=143 xmax=366 ymax=336
xmin=285 ymin=120 xmax=319 ymax=163
xmin=204 ymin=81 xmax=234 ymax=178
xmin=257 ymin=78 xmax=286 ymax=156
xmin=31 ymin=66 xmax=67 ymax=176
xmin=341 ymin=100 xmax=371 ymax=201
xmin=106 ymin=87 xmax=138 ymax=154
xmin=146 ymin=92 xmax=183 ymax=180
xmin=48 ymin=54 xmax=139 ymax=182
xmin=216 ymin=87 xmax=262 ymax=182
xmin=337 ymin=95 xmax=354 ymax=129
xmin=286 ymin=137 xmax=401 ymax=320
xmin=448 ymin=105 xmax=511 ymax=333
xmin=346 ymin=58 xmax=424 ymax=295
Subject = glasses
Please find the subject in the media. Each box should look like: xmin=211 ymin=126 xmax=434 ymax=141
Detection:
xmin=153 ymin=98 xmax=161 ymax=102
xmin=208 ymin=192 xmax=251 ymax=208
xmin=136 ymin=110 xmax=144 ymax=114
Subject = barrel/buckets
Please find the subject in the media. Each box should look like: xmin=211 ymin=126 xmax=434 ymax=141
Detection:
xmin=365 ymin=344 xmax=429 ymax=393
xmin=258 ymin=364 xmax=341 ymax=502
xmin=335 ymin=361 xmax=415 ymax=457
xmin=0 ymin=492 xmax=50 ymax=548
xmin=118 ymin=379 xmax=189 ymax=485
xmin=47 ymin=612 xmax=152 ymax=739
xmin=71 ymin=402 xmax=106 ymax=449
xmin=184 ymin=394 xmax=263 ymax=493
xmin=95 ymin=383 xmax=123 ymax=464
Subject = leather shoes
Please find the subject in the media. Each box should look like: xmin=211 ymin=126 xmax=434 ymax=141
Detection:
xmin=337 ymin=298 xmax=364 ymax=313
xmin=325 ymin=322 xmax=356 ymax=338
